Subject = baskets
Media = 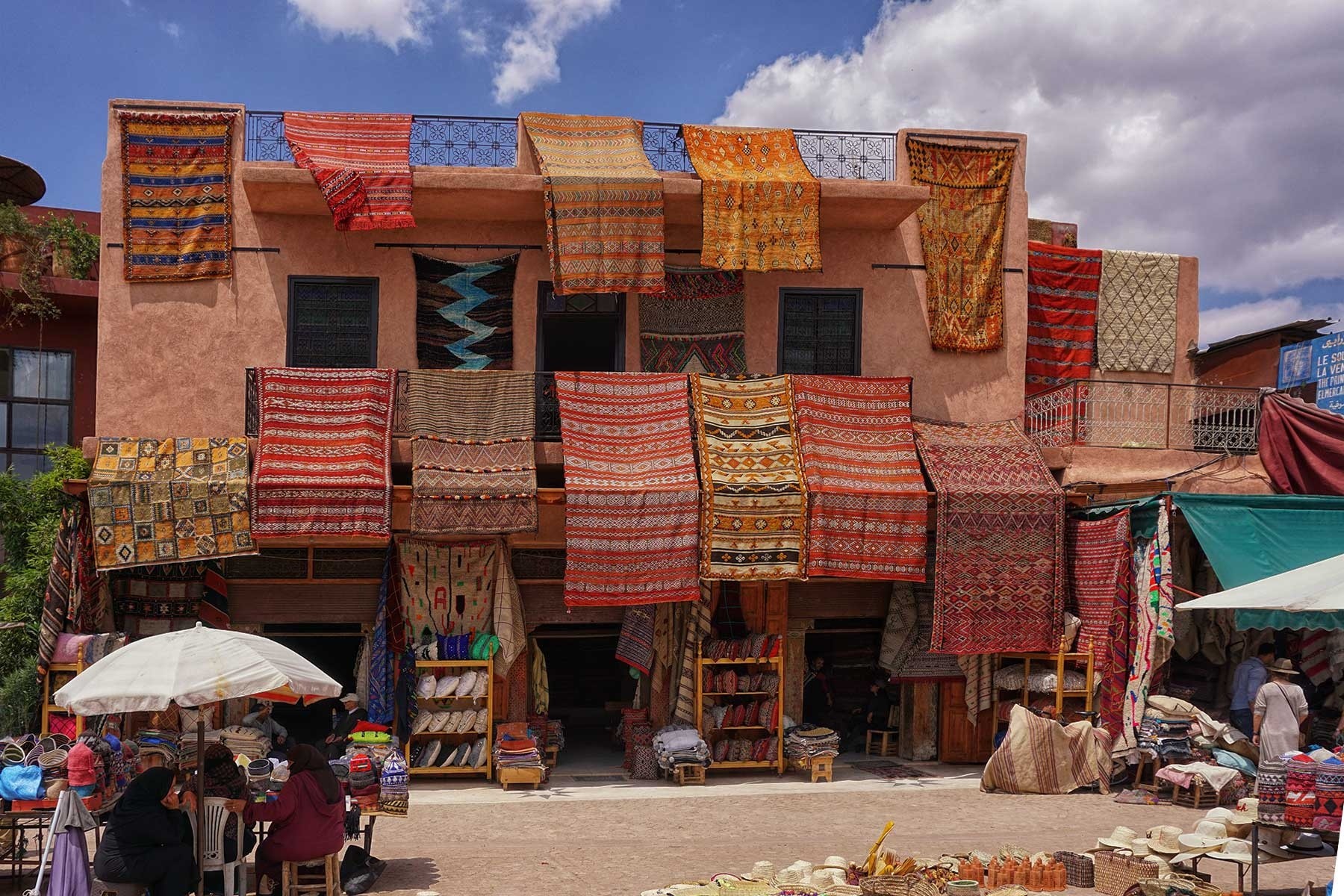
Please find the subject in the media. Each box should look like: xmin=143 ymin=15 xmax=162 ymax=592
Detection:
xmin=859 ymin=873 xmax=940 ymax=896
xmin=1137 ymin=872 xmax=1222 ymax=896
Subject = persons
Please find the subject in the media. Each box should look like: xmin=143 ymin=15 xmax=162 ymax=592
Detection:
xmin=224 ymin=744 xmax=347 ymax=896
xmin=1229 ymin=643 xmax=1274 ymax=744
xmin=241 ymin=703 xmax=293 ymax=762
xmin=806 ymin=656 xmax=832 ymax=723
xmin=94 ymin=766 xmax=202 ymax=896
xmin=178 ymin=744 xmax=257 ymax=864
xmin=316 ymin=693 xmax=367 ymax=761
xmin=1250 ymin=658 xmax=1309 ymax=796
xmin=844 ymin=681 xmax=887 ymax=754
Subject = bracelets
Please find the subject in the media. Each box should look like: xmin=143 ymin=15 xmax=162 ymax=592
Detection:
xmin=1253 ymin=731 xmax=1258 ymax=734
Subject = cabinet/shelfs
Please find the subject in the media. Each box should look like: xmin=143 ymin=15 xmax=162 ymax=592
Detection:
xmin=404 ymin=642 xmax=493 ymax=783
xmin=41 ymin=644 xmax=91 ymax=742
xmin=693 ymin=640 xmax=783 ymax=777
xmin=991 ymin=633 xmax=1094 ymax=757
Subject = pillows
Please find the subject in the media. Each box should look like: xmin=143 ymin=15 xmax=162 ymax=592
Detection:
xmin=624 ymin=708 xmax=660 ymax=780
xmin=52 ymin=630 xmax=131 ymax=663
xmin=701 ymin=633 xmax=779 ymax=763
xmin=147 ymin=703 xmax=263 ymax=740
xmin=411 ymin=629 xmax=527 ymax=769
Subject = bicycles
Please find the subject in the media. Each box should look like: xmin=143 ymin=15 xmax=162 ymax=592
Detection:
xmin=1021 ymin=704 xmax=1131 ymax=790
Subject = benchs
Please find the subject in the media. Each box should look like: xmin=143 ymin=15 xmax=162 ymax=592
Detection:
xmin=1132 ymin=751 xmax=1175 ymax=797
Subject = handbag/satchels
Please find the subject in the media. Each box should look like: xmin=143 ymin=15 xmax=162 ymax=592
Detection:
xmin=1053 ymin=850 xmax=1093 ymax=887
xmin=340 ymin=845 xmax=387 ymax=896
xmin=1094 ymin=848 xmax=1159 ymax=896
xmin=1298 ymin=731 xmax=1306 ymax=748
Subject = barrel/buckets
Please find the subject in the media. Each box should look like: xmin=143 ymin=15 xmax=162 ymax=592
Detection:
xmin=247 ymin=759 xmax=270 ymax=791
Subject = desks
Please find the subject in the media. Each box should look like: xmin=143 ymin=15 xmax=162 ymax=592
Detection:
xmin=0 ymin=799 xmax=376 ymax=896
xmin=1191 ymin=852 xmax=1315 ymax=892
xmin=1252 ymin=820 xmax=1340 ymax=896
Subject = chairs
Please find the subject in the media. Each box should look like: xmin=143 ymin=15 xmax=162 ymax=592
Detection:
xmin=187 ymin=797 xmax=247 ymax=896
xmin=866 ymin=705 xmax=899 ymax=757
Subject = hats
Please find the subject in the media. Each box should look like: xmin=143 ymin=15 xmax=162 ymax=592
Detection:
xmin=67 ymin=728 xmax=143 ymax=809
xmin=1078 ymin=797 xmax=1335 ymax=874
xmin=349 ymin=751 xmax=409 ymax=814
xmin=741 ymin=855 xmax=853 ymax=896
xmin=340 ymin=693 xmax=360 ymax=702
xmin=1265 ymin=658 xmax=1300 ymax=674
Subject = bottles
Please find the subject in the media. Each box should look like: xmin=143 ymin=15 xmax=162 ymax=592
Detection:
xmin=332 ymin=707 xmax=337 ymax=722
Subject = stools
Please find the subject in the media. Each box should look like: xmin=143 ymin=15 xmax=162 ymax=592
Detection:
xmin=545 ymin=746 xmax=560 ymax=768
xmin=811 ymin=756 xmax=833 ymax=782
xmin=90 ymin=877 xmax=143 ymax=896
xmin=674 ymin=763 xmax=706 ymax=785
xmin=496 ymin=768 xmax=541 ymax=790
xmin=282 ymin=852 xmax=340 ymax=896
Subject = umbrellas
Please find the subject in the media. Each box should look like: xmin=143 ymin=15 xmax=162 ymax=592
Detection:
xmin=52 ymin=621 xmax=342 ymax=896
xmin=1176 ymin=554 xmax=1344 ymax=613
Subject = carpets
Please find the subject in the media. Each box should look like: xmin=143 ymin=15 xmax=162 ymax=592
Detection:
xmin=846 ymin=760 xmax=943 ymax=778
xmin=1113 ymin=504 xmax=1174 ymax=752
xmin=572 ymin=775 xmax=627 ymax=783
xmin=1069 ymin=509 xmax=1132 ymax=744
xmin=87 ymin=112 xmax=1180 ymax=654
